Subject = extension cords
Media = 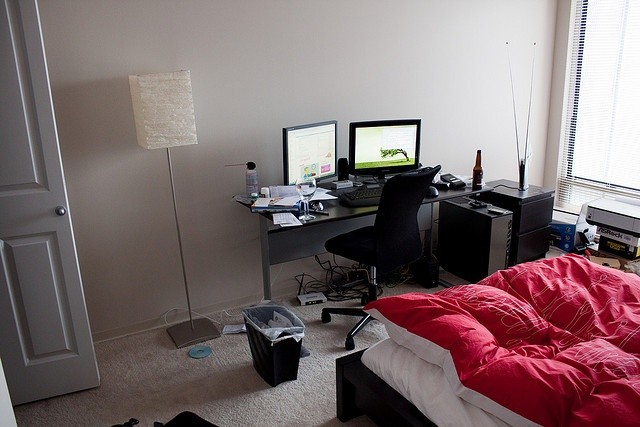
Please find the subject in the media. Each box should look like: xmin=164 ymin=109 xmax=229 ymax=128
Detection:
xmin=339 ymin=277 xmax=364 ymax=287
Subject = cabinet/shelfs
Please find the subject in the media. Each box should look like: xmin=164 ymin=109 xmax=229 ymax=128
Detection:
xmin=469 ymin=179 xmax=555 ymax=266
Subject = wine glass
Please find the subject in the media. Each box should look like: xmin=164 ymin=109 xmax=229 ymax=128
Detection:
xmin=295 ymin=177 xmax=316 ymax=220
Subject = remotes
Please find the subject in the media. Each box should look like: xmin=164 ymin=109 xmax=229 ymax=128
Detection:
xmin=488 ymin=208 xmax=504 ymax=215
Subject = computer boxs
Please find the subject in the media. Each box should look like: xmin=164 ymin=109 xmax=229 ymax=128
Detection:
xmin=438 ymin=196 xmax=514 ymax=281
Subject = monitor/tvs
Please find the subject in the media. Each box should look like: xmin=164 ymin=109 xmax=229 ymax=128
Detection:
xmin=283 ymin=119 xmax=337 ymax=186
xmin=348 ymin=119 xmax=422 ymax=184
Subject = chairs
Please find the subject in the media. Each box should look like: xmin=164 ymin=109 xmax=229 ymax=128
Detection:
xmin=321 ymin=164 xmax=441 ymax=350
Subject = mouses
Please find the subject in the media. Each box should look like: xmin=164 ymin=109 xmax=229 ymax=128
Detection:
xmin=311 ymin=200 xmax=325 ymax=211
xmin=426 ymin=186 xmax=439 ymax=198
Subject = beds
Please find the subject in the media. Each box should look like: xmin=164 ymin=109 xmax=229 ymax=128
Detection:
xmin=335 ymin=253 xmax=640 ymax=427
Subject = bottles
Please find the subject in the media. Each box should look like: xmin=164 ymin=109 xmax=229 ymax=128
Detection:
xmin=245 ymin=161 xmax=259 ymax=199
xmin=472 ymin=150 xmax=483 ymax=191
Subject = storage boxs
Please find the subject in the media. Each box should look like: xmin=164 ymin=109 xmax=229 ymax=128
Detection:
xmin=596 ymin=226 xmax=640 ymax=247
xmin=598 ymin=235 xmax=640 ymax=261
xmin=549 ymin=197 xmax=605 ymax=253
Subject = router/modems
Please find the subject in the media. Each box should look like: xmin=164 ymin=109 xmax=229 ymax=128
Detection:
xmin=296 ymin=272 xmax=327 ymax=306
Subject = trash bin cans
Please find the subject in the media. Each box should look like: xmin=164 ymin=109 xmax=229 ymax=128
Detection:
xmin=242 ymin=304 xmax=305 ymax=387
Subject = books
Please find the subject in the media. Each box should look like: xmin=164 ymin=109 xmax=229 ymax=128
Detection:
xmin=251 ymin=195 xmax=301 ymax=212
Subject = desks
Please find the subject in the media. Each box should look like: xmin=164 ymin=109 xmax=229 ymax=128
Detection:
xmin=232 ymin=185 xmax=494 ymax=301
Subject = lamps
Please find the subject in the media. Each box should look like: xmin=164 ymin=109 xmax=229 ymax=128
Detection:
xmin=128 ymin=69 xmax=222 ymax=350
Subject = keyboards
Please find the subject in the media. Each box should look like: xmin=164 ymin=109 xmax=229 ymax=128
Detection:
xmin=338 ymin=187 xmax=384 ymax=208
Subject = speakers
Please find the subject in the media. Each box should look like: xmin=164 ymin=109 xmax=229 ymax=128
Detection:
xmin=337 ymin=157 xmax=349 ymax=181
xmin=411 ymin=256 xmax=439 ymax=289
